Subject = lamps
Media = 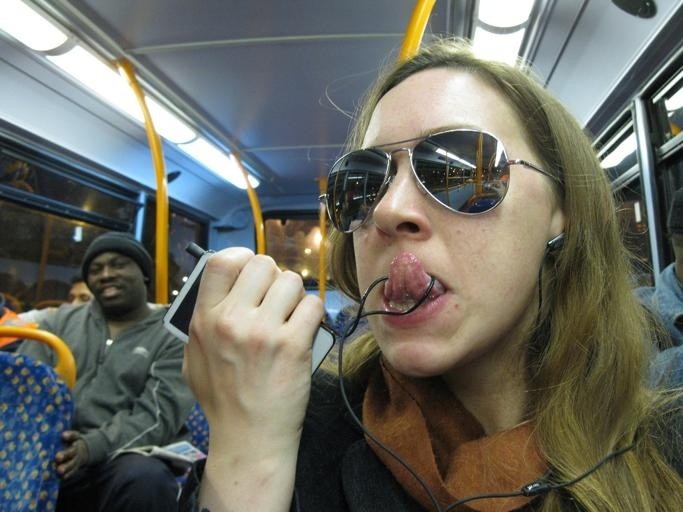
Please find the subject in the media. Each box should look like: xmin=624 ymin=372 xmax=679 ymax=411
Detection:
xmin=0 ymin=1 xmax=261 ymax=192
xmin=468 ymin=2 xmax=539 ymax=68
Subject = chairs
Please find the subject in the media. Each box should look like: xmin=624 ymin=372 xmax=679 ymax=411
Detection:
xmin=0 ymin=303 xmax=364 ymax=512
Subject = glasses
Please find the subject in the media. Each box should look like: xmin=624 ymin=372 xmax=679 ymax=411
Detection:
xmin=316 ymin=128 xmax=567 ymax=233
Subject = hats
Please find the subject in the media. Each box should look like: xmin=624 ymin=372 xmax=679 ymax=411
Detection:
xmin=79 ymin=230 xmax=153 ymax=287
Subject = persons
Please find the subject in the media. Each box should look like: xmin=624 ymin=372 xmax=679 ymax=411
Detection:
xmin=1 ymin=231 xmax=195 ymax=512
xmin=180 ymin=20 xmax=683 ymax=512
xmin=633 ymin=188 xmax=683 ymax=474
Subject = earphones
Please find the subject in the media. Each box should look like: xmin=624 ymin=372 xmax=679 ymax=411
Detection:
xmin=547 ymin=231 xmax=566 ymax=247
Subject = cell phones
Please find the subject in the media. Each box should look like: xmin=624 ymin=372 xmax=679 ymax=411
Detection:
xmin=161 ymin=241 xmax=337 ymax=379
